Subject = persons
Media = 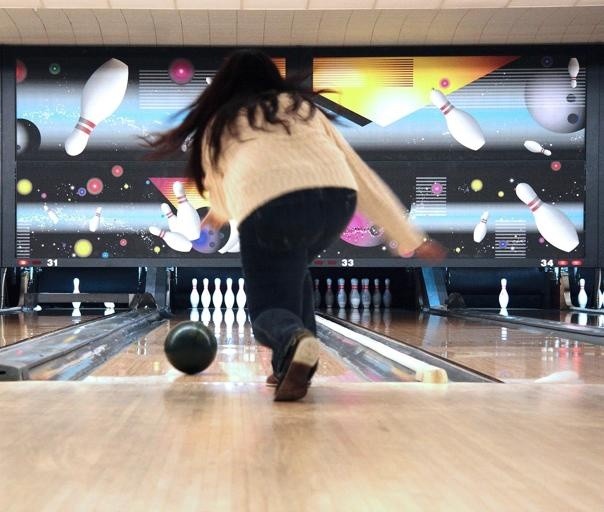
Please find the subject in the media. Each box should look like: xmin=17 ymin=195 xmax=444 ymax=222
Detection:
xmin=143 ymin=55 xmax=445 ymax=403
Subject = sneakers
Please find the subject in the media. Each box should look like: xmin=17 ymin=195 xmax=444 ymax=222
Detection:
xmin=265 ymin=373 xmax=310 ymax=387
xmin=273 ymin=328 xmax=319 ymax=402
